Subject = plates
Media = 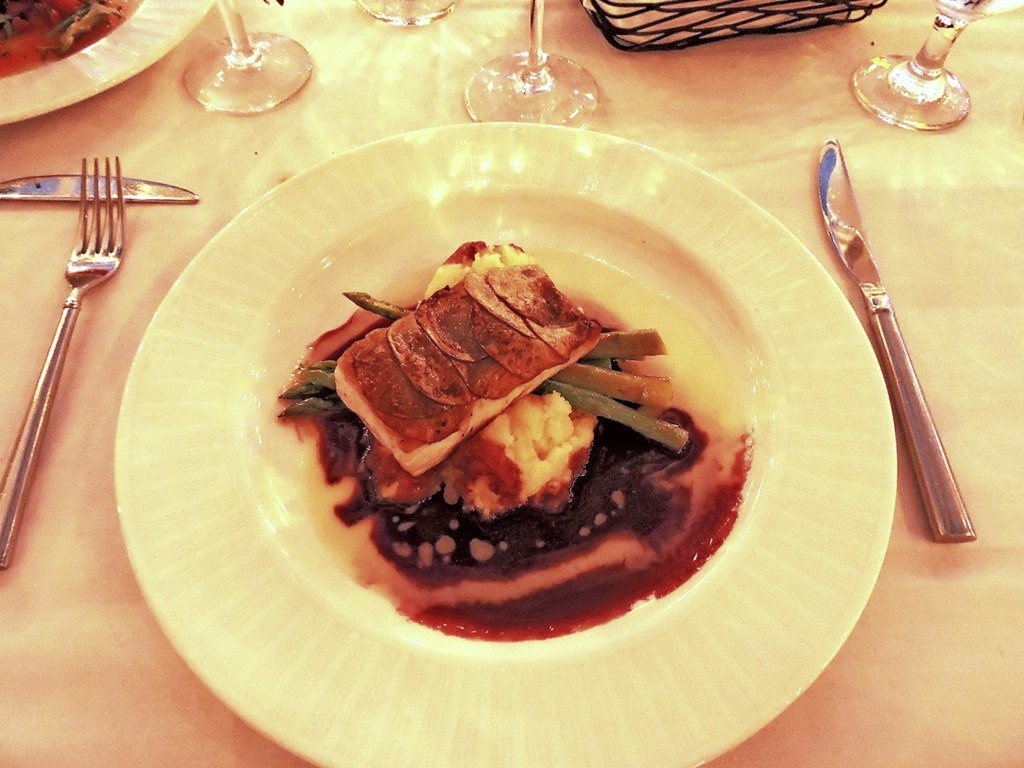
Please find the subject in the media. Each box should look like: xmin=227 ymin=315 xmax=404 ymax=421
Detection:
xmin=0 ymin=0 xmax=217 ymax=127
xmin=113 ymin=122 xmax=898 ymax=768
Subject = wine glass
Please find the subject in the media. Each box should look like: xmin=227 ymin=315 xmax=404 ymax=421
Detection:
xmin=849 ymin=0 xmax=1024 ymax=133
xmin=184 ymin=0 xmax=315 ymax=115
xmin=463 ymin=1 xmax=602 ymax=122
xmin=356 ymin=0 xmax=458 ymax=28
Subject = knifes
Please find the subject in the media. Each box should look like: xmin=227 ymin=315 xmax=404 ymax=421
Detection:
xmin=1 ymin=173 xmax=199 ymax=200
xmin=818 ymin=137 xmax=977 ymax=543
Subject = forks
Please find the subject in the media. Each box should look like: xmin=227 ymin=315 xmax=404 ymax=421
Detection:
xmin=0 ymin=156 xmax=126 ymax=572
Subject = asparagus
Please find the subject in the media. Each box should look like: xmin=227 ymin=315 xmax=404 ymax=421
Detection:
xmin=277 ymin=290 xmax=689 ymax=453
